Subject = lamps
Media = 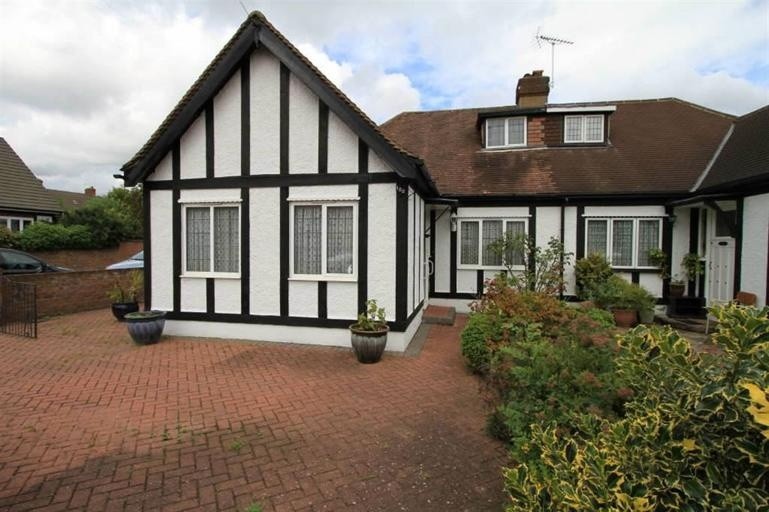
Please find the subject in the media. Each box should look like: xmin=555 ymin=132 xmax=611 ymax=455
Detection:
xmin=449 ymin=212 xmax=457 ymax=232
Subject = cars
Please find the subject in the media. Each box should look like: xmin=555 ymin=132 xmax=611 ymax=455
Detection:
xmin=0 ymin=247 xmax=76 ymax=275
xmin=106 ymin=250 xmax=145 ymax=270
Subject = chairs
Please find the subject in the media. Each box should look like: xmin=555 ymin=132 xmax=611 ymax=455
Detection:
xmin=705 ymin=291 xmax=755 ymax=334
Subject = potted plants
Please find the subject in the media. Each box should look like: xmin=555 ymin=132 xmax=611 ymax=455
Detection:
xmin=105 ymin=270 xmax=144 ymax=320
xmin=648 ymin=247 xmax=705 ymax=296
xmin=349 ymin=298 xmax=390 ymax=363
xmin=573 ymin=252 xmax=656 ymax=327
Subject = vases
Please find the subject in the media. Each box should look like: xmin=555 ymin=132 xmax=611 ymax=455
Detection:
xmin=126 ymin=310 xmax=166 ymax=344
